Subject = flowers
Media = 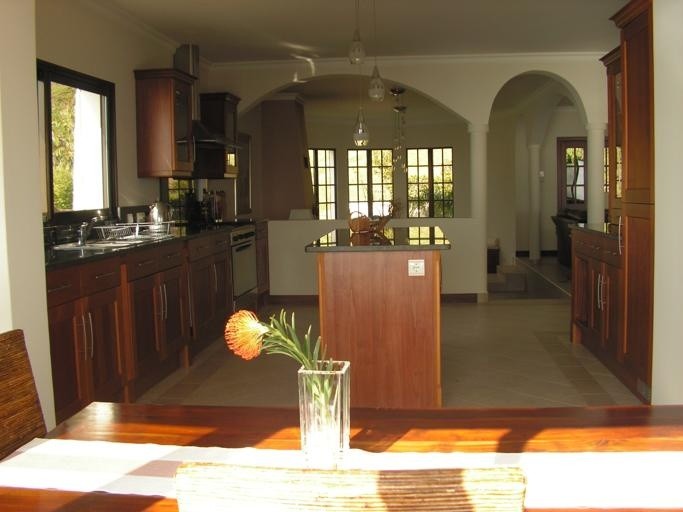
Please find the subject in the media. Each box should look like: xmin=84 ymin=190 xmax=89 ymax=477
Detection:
xmin=220 ymin=303 xmax=344 ymax=430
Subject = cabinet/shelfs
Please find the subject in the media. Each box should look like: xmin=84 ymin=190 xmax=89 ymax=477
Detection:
xmin=253 ymin=218 xmax=270 ymax=310
xmin=131 ymin=67 xmax=200 ymax=182
xmin=43 ymin=250 xmax=131 ymax=429
xmin=596 ymin=42 xmax=624 ymax=229
xmin=187 ymin=230 xmax=236 ymax=368
xmin=119 ymin=236 xmax=191 ymax=404
xmin=606 ymin=0 xmax=654 ymax=406
xmin=197 ymin=90 xmax=243 ymax=180
xmin=302 ymin=224 xmax=451 ymax=410
xmin=565 ymin=220 xmax=627 ymax=361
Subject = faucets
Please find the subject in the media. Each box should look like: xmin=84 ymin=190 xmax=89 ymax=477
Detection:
xmin=80 ymin=249 xmax=92 ymax=258
xmin=74 ymin=222 xmax=88 ymax=245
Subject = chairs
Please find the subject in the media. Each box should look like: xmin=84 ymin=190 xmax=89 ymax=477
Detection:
xmin=0 ymin=327 xmax=48 ymax=463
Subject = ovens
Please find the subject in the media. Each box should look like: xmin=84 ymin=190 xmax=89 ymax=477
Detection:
xmin=230 ymin=241 xmax=259 ymax=318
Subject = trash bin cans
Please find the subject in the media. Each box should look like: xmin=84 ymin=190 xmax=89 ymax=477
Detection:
xmin=487 ymin=248 xmax=500 ymax=273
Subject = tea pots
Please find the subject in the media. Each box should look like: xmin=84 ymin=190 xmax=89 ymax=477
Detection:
xmin=147 ymin=201 xmax=175 ymax=225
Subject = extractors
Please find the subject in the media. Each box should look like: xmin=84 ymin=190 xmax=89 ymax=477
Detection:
xmin=171 ymin=42 xmax=242 ymax=150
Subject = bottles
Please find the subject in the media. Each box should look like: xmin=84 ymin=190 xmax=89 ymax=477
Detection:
xmin=200 ymin=201 xmax=208 ymax=224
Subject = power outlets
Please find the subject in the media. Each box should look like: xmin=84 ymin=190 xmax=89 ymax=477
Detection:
xmin=407 ymin=259 xmax=424 ymax=277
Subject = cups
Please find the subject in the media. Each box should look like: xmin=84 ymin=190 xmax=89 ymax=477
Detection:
xmin=168 ymin=220 xmax=189 ymax=237
xmin=210 ymin=191 xmax=225 ymax=222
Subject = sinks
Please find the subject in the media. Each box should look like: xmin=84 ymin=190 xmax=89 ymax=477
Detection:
xmin=54 ymin=240 xmax=142 ymax=251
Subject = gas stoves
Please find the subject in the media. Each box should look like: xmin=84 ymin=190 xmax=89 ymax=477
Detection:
xmin=193 ymin=220 xmax=256 ymax=245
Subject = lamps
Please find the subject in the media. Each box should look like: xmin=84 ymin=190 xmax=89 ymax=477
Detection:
xmin=347 ymin=0 xmax=413 ymax=176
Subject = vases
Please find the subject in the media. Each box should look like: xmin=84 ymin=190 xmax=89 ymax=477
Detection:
xmin=292 ymin=357 xmax=351 ymax=475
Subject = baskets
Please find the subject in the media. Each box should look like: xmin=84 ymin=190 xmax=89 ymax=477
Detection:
xmin=347 ymin=211 xmax=371 ymax=233
